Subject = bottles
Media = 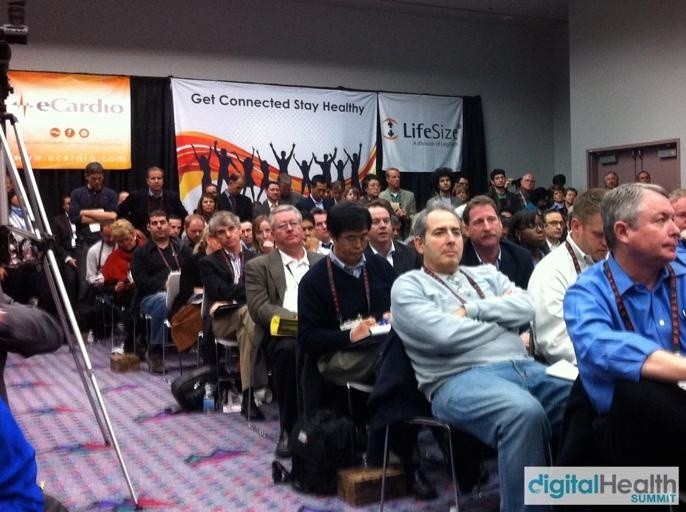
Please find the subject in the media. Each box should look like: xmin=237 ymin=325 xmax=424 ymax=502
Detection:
xmin=203 ymin=382 xmax=216 ymax=417
xmin=87 ymin=329 xmax=94 ymax=352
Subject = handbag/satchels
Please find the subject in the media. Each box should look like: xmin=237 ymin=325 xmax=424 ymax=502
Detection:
xmin=291 ymin=410 xmax=354 ymax=495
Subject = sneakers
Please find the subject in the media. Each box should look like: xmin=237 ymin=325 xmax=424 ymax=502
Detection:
xmin=149 ymin=353 xmax=167 ymax=374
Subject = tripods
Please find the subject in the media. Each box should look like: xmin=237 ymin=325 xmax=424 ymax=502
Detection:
xmin=0 ymin=101 xmax=142 ymax=512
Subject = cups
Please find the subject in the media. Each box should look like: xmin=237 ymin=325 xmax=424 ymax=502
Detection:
xmin=254 ymin=387 xmax=273 ymax=404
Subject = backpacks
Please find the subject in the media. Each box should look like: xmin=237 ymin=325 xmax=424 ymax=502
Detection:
xmin=171 ymin=367 xmax=234 ymax=410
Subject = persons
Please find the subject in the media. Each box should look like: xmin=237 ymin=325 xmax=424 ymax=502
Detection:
xmin=1 ymin=161 xmax=686 ymax=511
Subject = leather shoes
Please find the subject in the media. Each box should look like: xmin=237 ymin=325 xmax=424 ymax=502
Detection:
xmin=278 ymin=431 xmax=291 ymax=456
xmin=411 ymin=468 xmax=435 ymax=498
xmin=241 ymin=391 xmax=263 ymax=419
